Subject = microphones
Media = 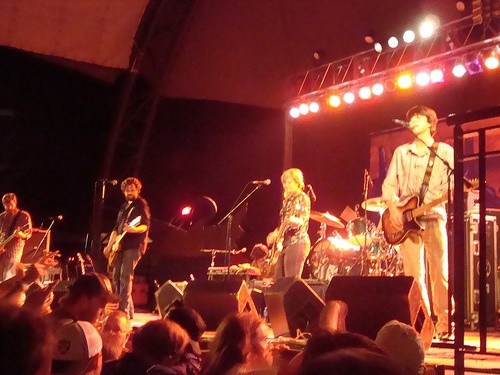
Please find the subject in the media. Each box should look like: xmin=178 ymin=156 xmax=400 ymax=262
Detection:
xmin=365 ymin=169 xmax=373 ymax=188
xmin=50 ymin=215 xmax=63 ymax=220
xmin=253 ymin=179 xmax=271 ymax=185
xmin=392 ymin=119 xmax=413 ymax=129
xmin=102 ymin=178 xmax=117 ymax=185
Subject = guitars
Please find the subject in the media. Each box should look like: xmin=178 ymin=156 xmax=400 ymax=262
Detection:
xmin=380 ymin=178 xmax=479 ymax=245
xmin=0 ymin=224 xmax=31 ymax=255
xmin=107 ymin=216 xmax=141 ymax=266
xmin=269 ymin=209 xmax=295 ymax=265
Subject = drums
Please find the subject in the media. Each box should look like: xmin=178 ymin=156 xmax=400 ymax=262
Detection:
xmin=346 ymin=217 xmax=375 ymax=244
xmin=307 ymin=236 xmax=361 ymax=282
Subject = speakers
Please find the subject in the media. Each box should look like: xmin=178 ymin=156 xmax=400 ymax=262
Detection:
xmin=328 ymin=276 xmax=436 ymax=355
xmin=154 ymin=280 xmax=258 ymax=332
xmin=263 ymin=277 xmax=326 ymax=338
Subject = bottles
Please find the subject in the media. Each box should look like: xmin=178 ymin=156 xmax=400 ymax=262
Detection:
xmin=263 ymin=307 xmax=270 ymax=324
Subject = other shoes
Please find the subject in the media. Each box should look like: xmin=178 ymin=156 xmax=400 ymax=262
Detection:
xmin=435 ymin=330 xmax=455 ymax=342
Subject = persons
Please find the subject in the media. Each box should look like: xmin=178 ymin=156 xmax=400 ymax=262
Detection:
xmin=250 ymin=242 xmax=271 ymax=277
xmin=0 ymin=192 xmax=31 ymax=283
xmin=103 ymin=178 xmax=150 ymax=322
xmin=0 ymin=251 xmax=428 ymax=375
xmin=380 ymin=105 xmax=457 ymax=342
xmin=267 ymin=168 xmax=313 ymax=282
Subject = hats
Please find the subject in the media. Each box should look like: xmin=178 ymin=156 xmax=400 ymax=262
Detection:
xmin=373 ymin=317 xmax=426 ymax=374
xmin=66 ymin=273 xmax=122 ymax=306
xmin=165 ymin=306 xmax=207 ymax=357
xmin=48 ymin=316 xmax=106 ymax=363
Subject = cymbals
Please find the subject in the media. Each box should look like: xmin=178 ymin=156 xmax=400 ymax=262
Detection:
xmin=362 ymin=196 xmax=389 ymax=212
xmin=309 ymin=210 xmax=346 ymax=229
